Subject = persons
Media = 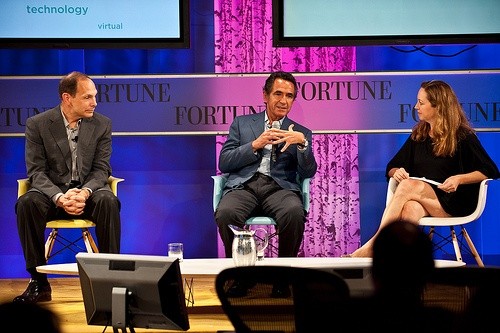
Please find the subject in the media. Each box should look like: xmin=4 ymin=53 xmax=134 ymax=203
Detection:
xmin=340 ymin=79 xmax=500 ymax=258
xmin=352 ymin=219 xmax=459 ymax=333
xmin=13 ymin=71 xmax=121 ymax=302
xmin=213 ymin=71 xmax=318 ymax=257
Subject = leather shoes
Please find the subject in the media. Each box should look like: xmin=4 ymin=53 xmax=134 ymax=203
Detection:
xmin=13 ymin=280 xmax=52 ymax=303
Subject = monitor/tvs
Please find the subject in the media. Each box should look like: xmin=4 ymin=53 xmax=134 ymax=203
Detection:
xmin=73 ymin=251 xmax=191 ymax=333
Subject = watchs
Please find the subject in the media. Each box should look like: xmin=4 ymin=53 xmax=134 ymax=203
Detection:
xmin=298 ymin=139 xmax=308 ymax=147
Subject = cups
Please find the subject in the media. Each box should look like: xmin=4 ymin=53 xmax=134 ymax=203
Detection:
xmin=168 ymin=243 xmax=183 ymax=263
xmin=258 ymin=246 xmax=264 ymax=261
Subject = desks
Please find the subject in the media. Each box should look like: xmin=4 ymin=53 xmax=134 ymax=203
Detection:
xmin=35 ymin=258 xmax=467 ymax=313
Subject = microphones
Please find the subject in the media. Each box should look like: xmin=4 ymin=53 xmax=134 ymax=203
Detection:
xmin=272 ymin=119 xmax=280 ymax=157
xmin=72 ymin=135 xmax=79 ymax=142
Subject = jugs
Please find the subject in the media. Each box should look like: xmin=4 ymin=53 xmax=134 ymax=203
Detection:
xmin=228 ymin=224 xmax=268 ymax=267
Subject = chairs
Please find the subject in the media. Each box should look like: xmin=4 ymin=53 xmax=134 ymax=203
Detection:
xmin=210 ymin=172 xmax=314 ymax=293
xmin=214 ymin=265 xmax=352 ymax=333
xmin=386 ymin=177 xmax=494 ymax=268
xmin=17 ymin=176 xmax=125 ymax=265
